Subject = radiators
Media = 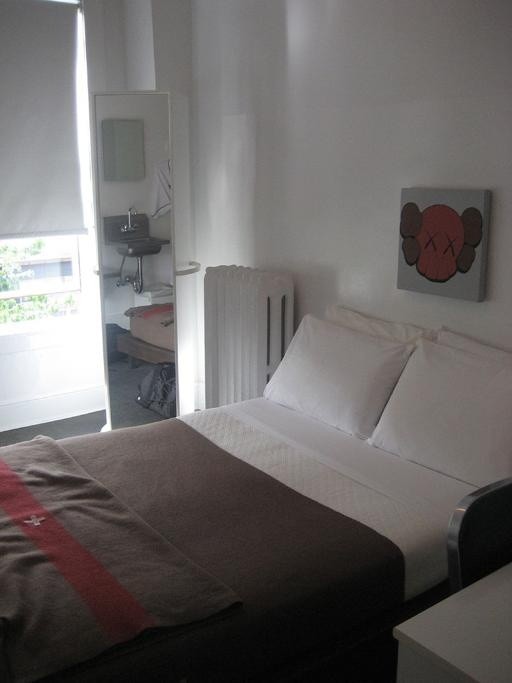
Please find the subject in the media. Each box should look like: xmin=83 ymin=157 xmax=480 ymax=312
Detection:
xmin=204 ymin=265 xmax=294 ymax=416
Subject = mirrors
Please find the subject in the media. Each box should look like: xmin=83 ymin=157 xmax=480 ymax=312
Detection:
xmin=101 ymin=117 xmax=143 ymax=184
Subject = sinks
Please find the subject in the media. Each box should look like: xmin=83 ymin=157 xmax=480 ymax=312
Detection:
xmin=117 ymin=236 xmax=171 ymax=256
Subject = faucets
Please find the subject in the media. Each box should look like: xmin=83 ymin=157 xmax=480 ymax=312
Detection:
xmin=120 ymin=205 xmax=140 ymax=235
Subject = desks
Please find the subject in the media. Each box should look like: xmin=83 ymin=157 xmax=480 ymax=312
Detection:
xmin=391 ymin=561 xmax=510 ymax=682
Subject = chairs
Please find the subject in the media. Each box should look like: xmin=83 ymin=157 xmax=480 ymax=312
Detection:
xmin=443 ymin=471 xmax=511 ymax=584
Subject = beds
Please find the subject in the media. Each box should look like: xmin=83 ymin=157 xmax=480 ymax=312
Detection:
xmin=0 ymin=302 xmax=510 ymax=683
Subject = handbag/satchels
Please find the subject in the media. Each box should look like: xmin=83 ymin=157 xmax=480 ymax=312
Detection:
xmin=134 ymin=361 xmax=176 ymax=418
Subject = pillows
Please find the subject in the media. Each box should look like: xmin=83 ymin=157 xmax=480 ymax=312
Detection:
xmin=433 ymin=325 xmax=512 ymax=369
xmin=320 ymin=305 xmax=434 ymax=365
xmin=370 ymin=336 xmax=511 ymax=487
xmin=263 ymin=313 xmax=416 ymax=441
